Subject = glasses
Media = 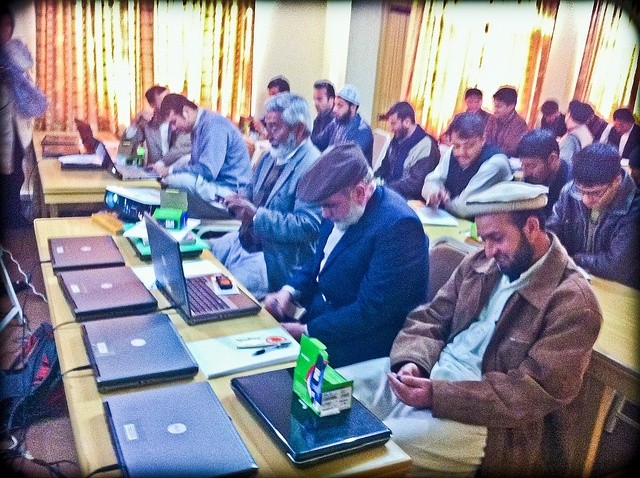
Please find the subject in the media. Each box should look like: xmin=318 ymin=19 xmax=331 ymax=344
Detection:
xmin=569 ymin=182 xmax=610 ymax=201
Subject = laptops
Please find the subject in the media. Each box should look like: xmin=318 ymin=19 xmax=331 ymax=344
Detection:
xmin=81 ymin=313 xmax=199 ymax=394
xmin=56 ymin=266 xmax=156 ymax=322
xmin=48 ymin=235 xmax=125 ymax=274
xmin=91 ymin=136 xmax=162 ymax=181
xmin=231 ymin=366 xmax=392 ymax=468
xmin=103 ymin=381 xmax=259 ymax=477
xmin=74 ymin=118 xmax=95 ymax=154
xmin=144 ymin=211 xmax=262 ymax=326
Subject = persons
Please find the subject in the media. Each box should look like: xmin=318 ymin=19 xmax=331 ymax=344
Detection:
xmin=607 ymin=108 xmax=639 ymax=171
xmin=310 ymin=80 xmax=336 ymax=148
xmin=373 ymin=100 xmax=440 ymax=203
xmin=484 ymin=86 xmax=525 ymax=155
xmin=329 ymin=83 xmax=377 ymax=164
xmin=628 ymin=143 xmax=639 ymax=179
xmin=543 ymin=140 xmax=640 ymax=288
xmin=539 ymin=100 xmax=565 ymax=133
xmin=210 ymin=91 xmax=327 ymax=298
xmin=592 ymin=104 xmax=609 ymax=142
xmin=253 ymin=77 xmax=290 ymax=145
xmin=263 ymin=140 xmax=432 ymax=370
xmin=557 ymin=99 xmax=592 ymax=181
xmin=0 ymin=10 xmax=33 ymax=249
xmin=122 ymin=85 xmax=193 ymax=173
xmin=158 ymin=94 xmax=250 ymax=217
xmin=508 ymin=127 xmax=574 ymax=227
xmin=334 ymin=181 xmax=605 ymax=478
xmin=441 ymin=88 xmax=492 ymax=137
xmin=421 ymin=113 xmax=515 ymax=225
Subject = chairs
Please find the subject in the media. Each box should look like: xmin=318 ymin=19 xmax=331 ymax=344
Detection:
xmin=371 ymin=130 xmax=388 ymax=173
xmin=436 ymin=143 xmax=452 ymax=180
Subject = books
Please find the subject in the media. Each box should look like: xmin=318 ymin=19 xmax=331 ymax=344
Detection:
xmin=123 ymin=215 xmax=197 ymax=246
xmin=40 ymin=133 xmax=81 ymax=158
xmin=185 ymin=326 xmax=301 ymax=382
xmin=411 ymin=206 xmax=459 ymax=226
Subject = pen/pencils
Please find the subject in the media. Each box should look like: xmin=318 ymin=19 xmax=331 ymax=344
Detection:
xmin=459 ymin=227 xmax=471 ymax=233
xmin=252 ymin=341 xmax=291 ymax=356
xmin=237 ymin=343 xmax=278 ymax=349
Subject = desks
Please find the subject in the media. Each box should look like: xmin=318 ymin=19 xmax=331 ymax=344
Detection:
xmin=30 ymin=126 xmax=162 ymax=211
xmin=401 ymin=195 xmax=636 ymax=393
xmin=30 ymin=214 xmax=412 ymax=476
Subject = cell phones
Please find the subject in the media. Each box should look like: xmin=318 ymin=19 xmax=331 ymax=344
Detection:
xmin=215 ymin=194 xmax=225 ymax=200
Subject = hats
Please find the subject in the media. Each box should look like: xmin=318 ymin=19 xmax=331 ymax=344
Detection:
xmin=295 ymin=142 xmax=367 ymax=202
xmin=336 ymin=84 xmax=359 ymax=106
xmin=464 ymin=181 xmax=549 ymax=216
